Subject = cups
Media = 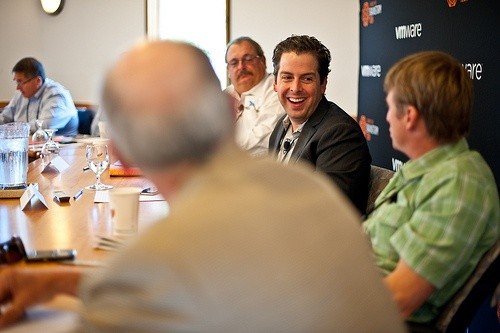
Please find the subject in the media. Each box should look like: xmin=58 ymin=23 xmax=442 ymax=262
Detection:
xmin=97 ymin=121 xmax=108 ymax=141
xmin=109 ymin=187 xmax=141 ymax=236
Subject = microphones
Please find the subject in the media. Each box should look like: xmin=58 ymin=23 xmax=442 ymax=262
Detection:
xmin=390 ymin=193 xmax=398 ymax=202
xmin=283 ymin=141 xmax=290 ymax=148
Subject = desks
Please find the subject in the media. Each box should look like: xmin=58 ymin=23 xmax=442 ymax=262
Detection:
xmin=0 ymin=135 xmax=171 ymax=333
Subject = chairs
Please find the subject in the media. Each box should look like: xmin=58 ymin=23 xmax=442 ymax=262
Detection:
xmin=415 ymin=243 xmax=500 ymax=332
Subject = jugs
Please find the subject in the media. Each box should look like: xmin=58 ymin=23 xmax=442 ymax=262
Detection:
xmin=0 ymin=121 xmax=31 ymax=189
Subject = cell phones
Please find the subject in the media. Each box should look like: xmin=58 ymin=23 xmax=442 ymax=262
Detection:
xmin=26 ymin=249 xmax=75 ymax=260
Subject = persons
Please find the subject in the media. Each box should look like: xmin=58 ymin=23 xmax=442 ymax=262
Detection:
xmin=269 ymin=35 xmax=373 ymax=218
xmin=0 ymin=39 xmax=407 ymax=333
xmin=0 ymin=57 xmax=78 ymax=137
xmin=222 ymin=37 xmax=286 ymax=151
xmin=361 ymin=53 xmax=500 ymax=333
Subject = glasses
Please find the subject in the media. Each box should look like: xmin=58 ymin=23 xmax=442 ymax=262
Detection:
xmin=228 ymin=55 xmax=260 ymax=68
xmin=14 ymin=76 xmax=37 ymax=86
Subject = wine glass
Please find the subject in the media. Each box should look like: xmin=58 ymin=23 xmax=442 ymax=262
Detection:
xmin=32 ymin=119 xmax=50 ymax=151
xmin=40 ymin=129 xmax=60 ymax=165
xmin=85 ymin=145 xmax=114 ymax=190
